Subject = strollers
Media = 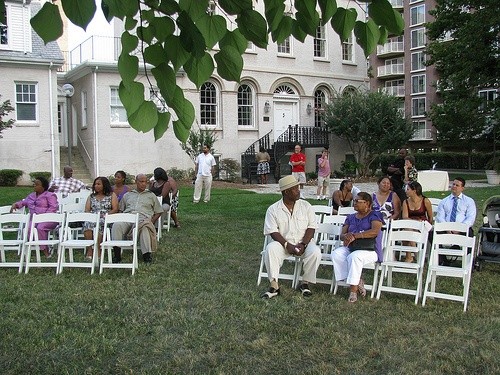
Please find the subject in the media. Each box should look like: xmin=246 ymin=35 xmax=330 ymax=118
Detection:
xmin=473 ymin=194 xmax=500 ymax=272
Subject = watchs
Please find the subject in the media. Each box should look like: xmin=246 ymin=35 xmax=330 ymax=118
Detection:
xmin=299 ymin=241 xmax=307 ymax=249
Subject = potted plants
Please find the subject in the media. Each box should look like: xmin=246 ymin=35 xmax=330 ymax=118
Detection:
xmin=484 ymin=156 xmax=500 ymax=185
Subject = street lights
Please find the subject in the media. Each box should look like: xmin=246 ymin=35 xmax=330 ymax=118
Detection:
xmin=61 ymin=84 xmax=75 ymax=167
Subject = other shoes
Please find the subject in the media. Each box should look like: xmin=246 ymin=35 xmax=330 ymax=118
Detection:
xmin=86 ymin=255 xmax=92 ymax=260
xmin=299 ymin=284 xmax=312 ymax=297
xmin=404 ymin=256 xmax=413 ymax=263
xmin=261 ymin=286 xmax=280 ymax=298
xmin=300 ymin=195 xmax=305 ymax=200
xmin=440 ymin=258 xmax=444 ymax=266
xmin=323 ymin=195 xmax=326 ymax=200
xmin=316 ymin=196 xmax=321 ymax=200
xmin=144 ymin=253 xmax=152 ymax=263
xmin=112 ymin=257 xmax=121 ymax=262
xmin=176 ymin=223 xmax=180 ymax=228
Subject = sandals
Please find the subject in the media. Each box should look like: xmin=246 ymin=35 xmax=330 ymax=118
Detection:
xmin=348 ymin=291 xmax=357 ymax=302
xmin=358 ymin=279 xmax=366 ymax=297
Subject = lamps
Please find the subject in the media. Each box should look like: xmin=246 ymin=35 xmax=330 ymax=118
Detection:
xmin=306 ymin=103 xmax=313 ymax=114
xmin=263 ymin=100 xmax=271 ymax=113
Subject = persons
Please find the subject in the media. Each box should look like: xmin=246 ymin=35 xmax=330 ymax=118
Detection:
xmin=150 ymin=168 xmax=170 ymax=218
xmin=332 ymin=179 xmax=354 ymax=215
xmin=81 ymin=177 xmax=119 ymax=259
xmin=317 ymin=149 xmax=331 ymax=201
xmin=11 ymin=176 xmax=59 ymax=258
xmin=291 ymin=145 xmax=306 ymax=189
xmin=401 ymin=182 xmax=433 ymax=263
xmin=369 ymin=176 xmax=401 ymax=261
xmin=109 ymin=171 xmax=130 ymax=203
xmin=428 ymin=177 xmax=477 ymax=272
xmin=256 ymin=147 xmax=270 ymax=184
xmin=402 ymin=156 xmax=418 ymax=200
xmin=48 ymin=166 xmax=92 ymax=200
xmin=387 ymin=147 xmax=408 ymax=202
xmin=333 ymin=192 xmax=386 ymax=303
xmin=261 ymin=175 xmax=322 ymax=299
xmin=193 ymin=144 xmax=216 ymax=205
xmin=167 ymin=176 xmax=180 ymax=228
xmin=111 ymin=174 xmax=163 ymax=265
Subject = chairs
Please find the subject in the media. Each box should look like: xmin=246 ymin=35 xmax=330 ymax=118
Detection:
xmin=0 ymin=188 xmax=180 ymax=277
xmin=256 ymin=198 xmax=477 ymax=312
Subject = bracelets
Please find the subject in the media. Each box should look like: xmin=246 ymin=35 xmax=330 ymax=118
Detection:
xmin=352 ymin=233 xmax=356 ymax=240
xmin=360 ymin=231 xmax=363 ymax=239
xmin=284 ymin=241 xmax=289 ymax=250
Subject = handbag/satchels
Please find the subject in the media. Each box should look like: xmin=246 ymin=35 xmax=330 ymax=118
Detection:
xmin=349 ymin=237 xmax=375 ymax=250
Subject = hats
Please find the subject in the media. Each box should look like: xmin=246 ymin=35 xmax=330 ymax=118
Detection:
xmin=277 ymin=175 xmax=301 ymax=192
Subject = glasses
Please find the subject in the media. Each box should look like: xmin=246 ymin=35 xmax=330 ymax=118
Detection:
xmin=354 ymin=198 xmax=369 ymax=203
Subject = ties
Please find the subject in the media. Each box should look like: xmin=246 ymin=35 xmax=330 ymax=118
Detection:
xmin=450 ymin=197 xmax=458 ymax=223
